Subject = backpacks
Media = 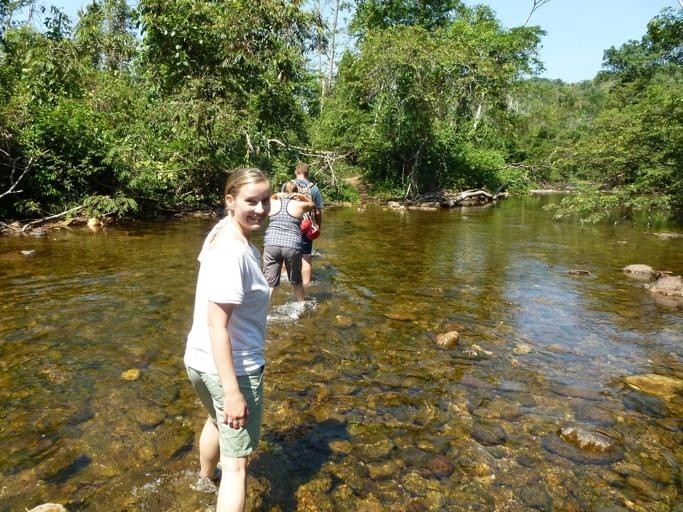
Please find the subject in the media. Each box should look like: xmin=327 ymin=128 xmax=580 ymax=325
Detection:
xmin=291 ymin=180 xmax=315 ymax=221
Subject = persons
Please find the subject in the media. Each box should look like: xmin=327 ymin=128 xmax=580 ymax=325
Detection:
xmin=281 ymin=163 xmax=322 ymax=280
xmin=262 ymin=181 xmax=315 ymax=301
xmin=183 ymin=168 xmax=270 ymax=512
